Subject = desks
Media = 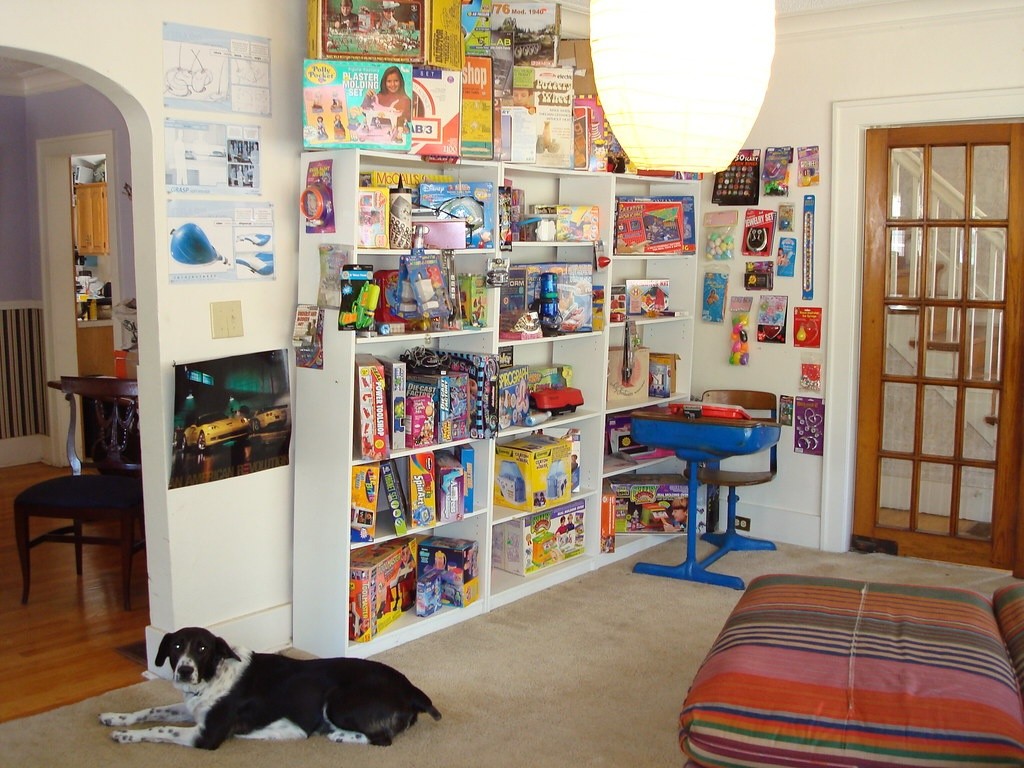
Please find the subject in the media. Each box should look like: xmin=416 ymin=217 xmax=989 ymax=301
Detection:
xmin=631 ymin=410 xmax=776 ymax=593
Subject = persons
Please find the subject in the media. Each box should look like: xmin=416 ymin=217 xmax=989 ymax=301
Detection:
xmin=556 ymin=514 xmax=574 ymax=536
xmin=571 ymin=454 xmax=578 ymax=471
xmin=663 ymin=497 xmax=690 ymax=533
xmin=534 ymin=492 xmax=546 ymax=507
xmin=334 ymin=0 xmax=401 ymax=34
xmin=361 ymin=65 xmax=411 ymax=127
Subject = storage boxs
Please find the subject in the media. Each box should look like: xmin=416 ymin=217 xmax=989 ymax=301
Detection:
xmin=302 ymin=1 xmax=701 ymax=645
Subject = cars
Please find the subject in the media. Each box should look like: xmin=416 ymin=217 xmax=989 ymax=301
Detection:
xmin=182 ymin=411 xmax=253 ymax=452
xmin=249 ymin=406 xmax=288 ymax=433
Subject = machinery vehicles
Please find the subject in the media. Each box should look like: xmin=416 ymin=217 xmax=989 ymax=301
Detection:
xmin=498 ymin=17 xmax=541 ymax=59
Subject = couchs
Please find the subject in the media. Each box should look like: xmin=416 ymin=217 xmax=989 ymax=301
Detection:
xmin=677 ymin=574 xmax=1022 ymax=767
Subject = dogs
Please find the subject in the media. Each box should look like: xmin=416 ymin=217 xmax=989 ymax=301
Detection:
xmin=97 ymin=627 xmax=442 ymax=750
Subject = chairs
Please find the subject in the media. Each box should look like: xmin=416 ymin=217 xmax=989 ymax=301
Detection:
xmin=13 ymin=375 xmax=141 ymax=611
xmin=700 ymin=390 xmax=779 ymax=552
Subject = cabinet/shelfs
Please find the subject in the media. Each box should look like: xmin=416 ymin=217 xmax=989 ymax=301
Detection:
xmin=292 ymin=146 xmax=701 ymax=660
xmin=78 ymin=325 xmax=115 ymax=376
xmin=74 ymin=181 xmax=108 ymax=255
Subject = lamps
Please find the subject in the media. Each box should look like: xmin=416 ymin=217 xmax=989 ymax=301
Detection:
xmin=589 ymin=1 xmax=778 ymax=176
